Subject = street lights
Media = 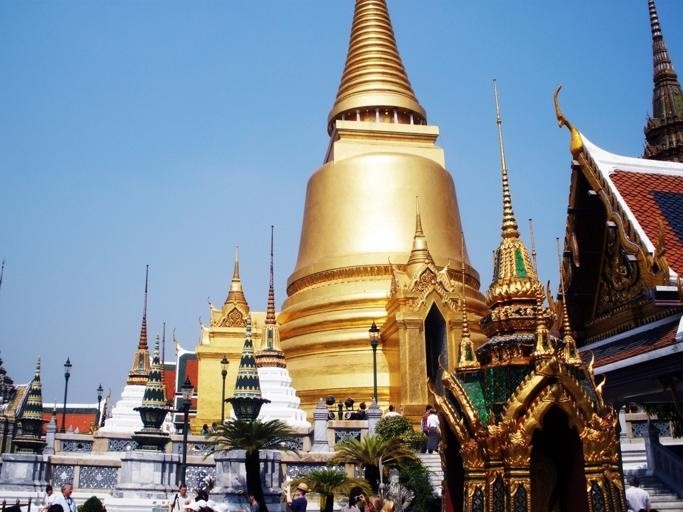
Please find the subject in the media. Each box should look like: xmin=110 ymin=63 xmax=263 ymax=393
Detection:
xmin=94 ymin=383 xmax=105 ymax=426
xmin=219 ymin=353 xmax=230 ymax=424
xmin=367 ymin=318 xmax=383 ymax=407
xmin=58 ymin=356 xmax=73 ymax=434
xmin=180 ymin=375 xmax=196 ymax=488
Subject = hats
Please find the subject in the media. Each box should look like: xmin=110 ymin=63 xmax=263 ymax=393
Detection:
xmin=296 ymin=481 xmax=311 ymax=493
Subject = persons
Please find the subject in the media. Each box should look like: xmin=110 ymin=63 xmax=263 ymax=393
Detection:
xmin=427 ymin=409 xmax=442 ymax=453
xmin=387 ymin=403 xmax=401 ymax=417
xmin=359 ymin=400 xmax=367 ymax=419
xmin=420 ymin=404 xmax=438 ymax=453
xmin=47 ymin=504 xmax=64 ymax=512
xmin=167 ymin=481 xmax=397 ymax=512
xmin=624 ymin=477 xmax=651 ymax=512
xmin=38 ymin=484 xmax=56 ymax=512
xmin=210 ymin=421 xmax=218 ymax=433
xmin=201 ymin=424 xmax=210 ymax=433
xmin=51 ymin=483 xmax=77 ymax=512
